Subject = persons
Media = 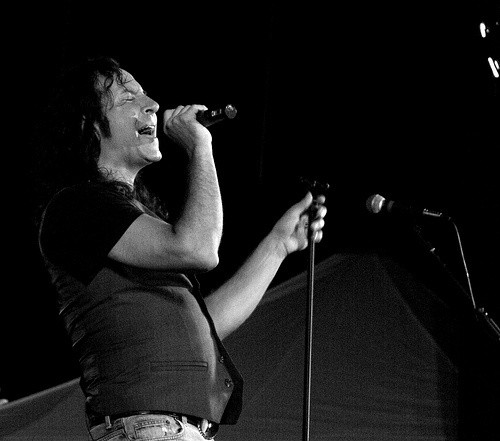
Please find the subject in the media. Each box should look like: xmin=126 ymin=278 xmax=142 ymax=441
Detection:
xmin=30 ymin=52 xmax=327 ymax=441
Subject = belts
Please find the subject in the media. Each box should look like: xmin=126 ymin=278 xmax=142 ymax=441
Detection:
xmin=88 ymin=410 xmax=214 ymax=436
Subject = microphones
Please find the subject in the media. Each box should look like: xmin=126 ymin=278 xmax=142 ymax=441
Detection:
xmin=367 ymin=193 xmax=452 ymax=220
xmin=196 ymin=105 xmax=237 ymax=128
